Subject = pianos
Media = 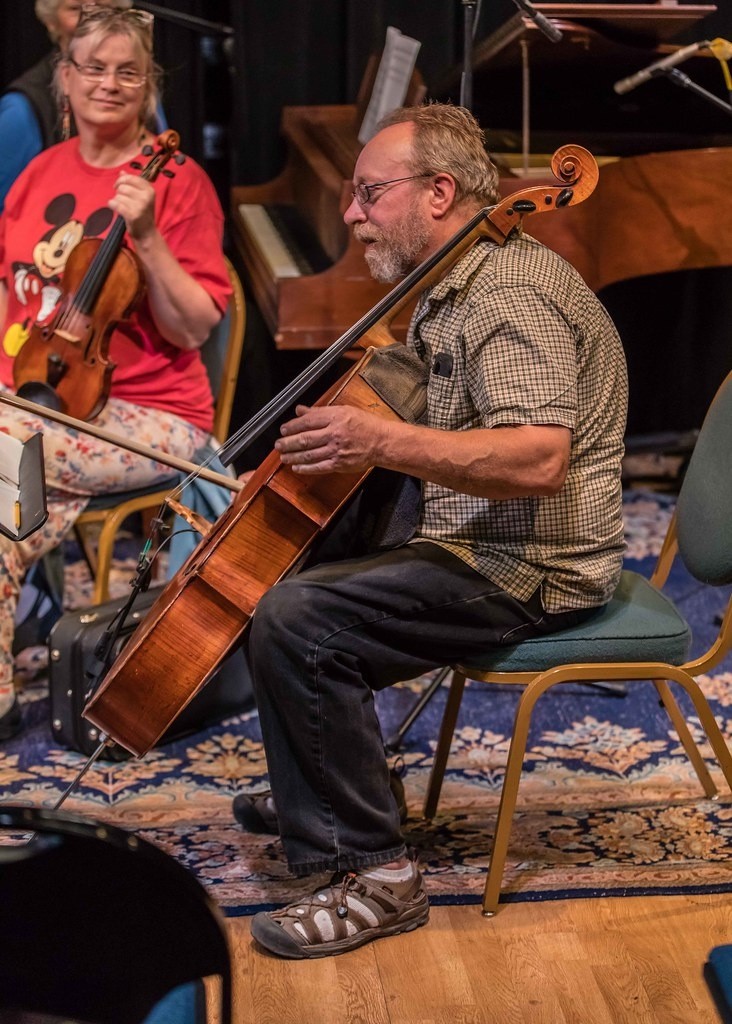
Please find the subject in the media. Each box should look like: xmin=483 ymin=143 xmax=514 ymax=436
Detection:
xmin=232 ymin=48 xmax=732 ymax=352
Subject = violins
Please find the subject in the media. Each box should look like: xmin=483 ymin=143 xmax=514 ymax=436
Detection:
xmin=12 ymin=129 xmax=187 ymax=421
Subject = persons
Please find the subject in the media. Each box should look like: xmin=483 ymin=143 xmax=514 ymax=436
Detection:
xmin=0 ymin=6 xmax=239 ymax=721
xmin=231 ymin=103 xmax=629 ymax=958
xmin=1 ymin=0 xmax=170 ymax=217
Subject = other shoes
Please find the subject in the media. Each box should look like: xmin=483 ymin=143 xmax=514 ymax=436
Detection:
xmin=0 ymin=696 xmax=22 ymax=739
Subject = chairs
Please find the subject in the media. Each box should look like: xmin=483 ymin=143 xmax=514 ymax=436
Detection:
xmin=63 ymin=252 xmax=246 ymax=608
xmin=422 ymin=371 xmax=732 ymax=917
xmin=0 ymin=802 xmax=234 ymax=1024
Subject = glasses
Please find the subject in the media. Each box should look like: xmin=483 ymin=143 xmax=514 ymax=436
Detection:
xmin=352 ymin=172 xmax=462 ymax=205
xmin=70 ymin=57 xmax=146 ymax=87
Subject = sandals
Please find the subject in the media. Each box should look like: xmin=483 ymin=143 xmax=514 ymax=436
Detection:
xmin=252 ymin=862 xmax=430 ymax=958
xmin=233 ymin=790 xmax=278 ymax=837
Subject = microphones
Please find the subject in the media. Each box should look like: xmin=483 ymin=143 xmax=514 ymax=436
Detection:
xmin=614 ymin=41 xmax=709 ymax=94
xmin=512 ymin=0 xmax=563 ymax=42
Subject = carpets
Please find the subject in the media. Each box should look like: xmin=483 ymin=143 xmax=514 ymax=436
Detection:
xmin=0 ymin=423 xmax=732 ymax=918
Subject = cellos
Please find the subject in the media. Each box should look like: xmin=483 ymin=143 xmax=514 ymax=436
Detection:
xmin=48 ymin=141 xmax=599 ymax=814
